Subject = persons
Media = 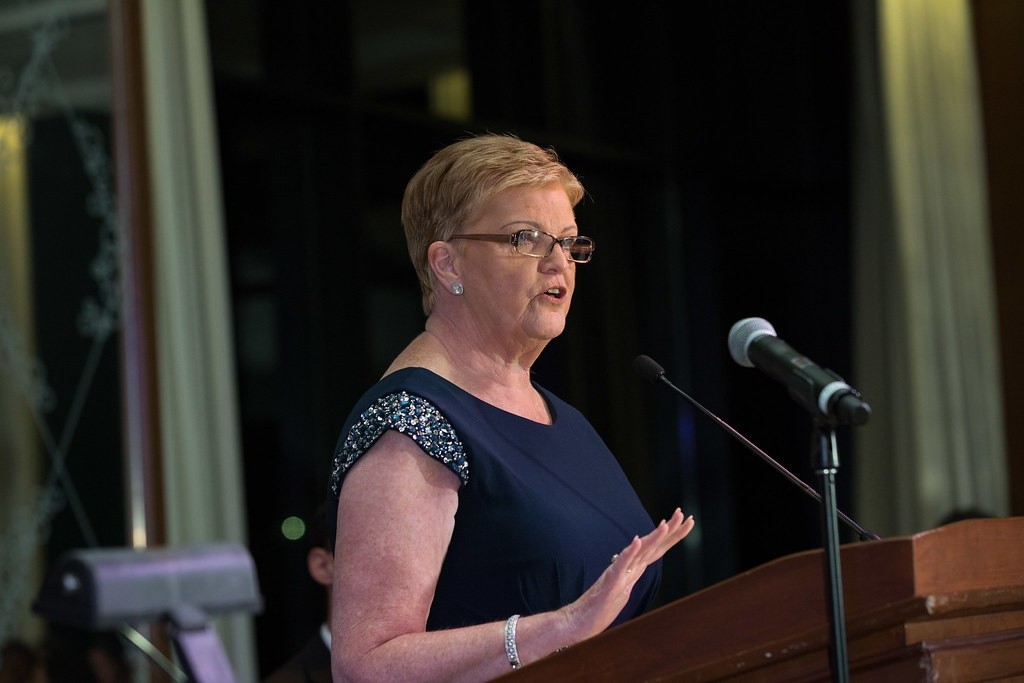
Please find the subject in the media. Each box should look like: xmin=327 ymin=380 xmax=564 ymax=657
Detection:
xmin=262 ymin=503 xmax=337 ymax=683
xmin=331 ymin=136 xmax=695 ymax=683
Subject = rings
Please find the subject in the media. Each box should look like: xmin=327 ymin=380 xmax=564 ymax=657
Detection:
xmin=611 ymin=554 xmax=631 ymax=573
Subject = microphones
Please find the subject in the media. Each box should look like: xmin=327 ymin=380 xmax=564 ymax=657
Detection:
xmin=728 ymin=317 xmax=871 ymax=425
xmin=630 ymin=354 xmax=882 ymax=544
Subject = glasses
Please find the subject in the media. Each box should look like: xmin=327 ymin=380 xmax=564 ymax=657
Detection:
xmin=446 ymin=229 xmax=595 ymax=263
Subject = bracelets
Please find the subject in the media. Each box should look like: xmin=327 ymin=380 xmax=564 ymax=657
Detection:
xmin=504 ymin=615 xmax=522 ymax=670
